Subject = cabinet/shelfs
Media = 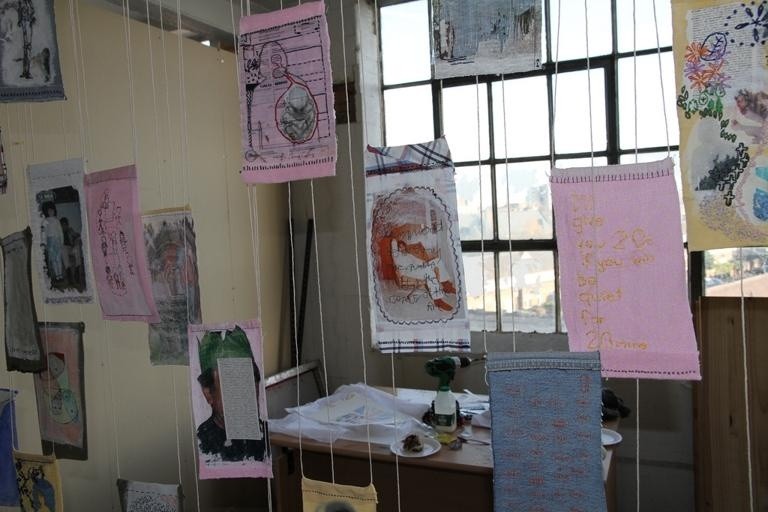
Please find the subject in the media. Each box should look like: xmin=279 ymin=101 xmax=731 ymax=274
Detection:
xmin=693 ymin=296 xmax=768 ymax=512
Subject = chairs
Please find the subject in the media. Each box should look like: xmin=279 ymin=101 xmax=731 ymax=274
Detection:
xmin=265 ymin=359 xmax=331 ymax=476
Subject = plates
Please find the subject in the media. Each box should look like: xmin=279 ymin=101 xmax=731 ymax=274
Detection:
xmin=600 ymin=427 xmax=623 ymax=445
xmin=389 ymin=437 xmax=441 ymax=458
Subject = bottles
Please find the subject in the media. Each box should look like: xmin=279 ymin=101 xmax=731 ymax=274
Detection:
xmin=434 ymin=386 xmax=457 ymax=432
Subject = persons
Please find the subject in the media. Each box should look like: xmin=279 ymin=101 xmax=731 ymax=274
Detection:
xmin=196 ymin=327 xmax=270 ymax=463
xmin=38 ymin=204 xmax=65 ymax=282
xmin=392 ymin=242 xmax=457 ymax=310
xmin=61 ymin=215 xmax=86 ymax=280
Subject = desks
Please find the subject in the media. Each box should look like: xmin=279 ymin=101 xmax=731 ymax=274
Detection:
xmin=267 ymin=386 xmax=621 ymax=512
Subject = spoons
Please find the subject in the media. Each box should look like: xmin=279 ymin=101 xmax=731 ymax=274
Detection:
xmin=456 ymin=435 xmax=490 ymax=445
